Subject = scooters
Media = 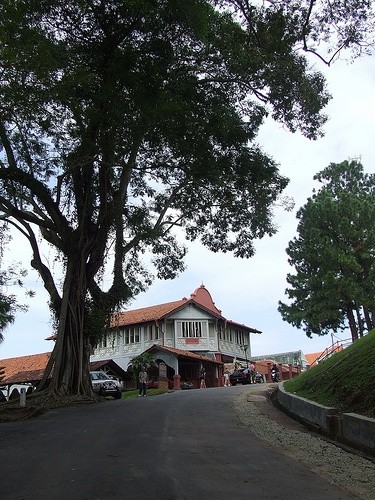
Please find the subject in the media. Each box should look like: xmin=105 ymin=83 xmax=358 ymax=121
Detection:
xmin=271 ymin=369 xmax=280 ymax=383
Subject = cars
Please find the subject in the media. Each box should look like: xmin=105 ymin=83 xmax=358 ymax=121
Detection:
xmin=146 ymin=377 xmax=175 ymax=389
xmin=170 ymin=377 xmax=194 ymax=389
xmin=229 ymin=369 xmax=264 ymax=386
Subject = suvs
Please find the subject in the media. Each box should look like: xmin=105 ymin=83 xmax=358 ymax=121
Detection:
xmin=90 ymin=370 xmax=123 ymax=399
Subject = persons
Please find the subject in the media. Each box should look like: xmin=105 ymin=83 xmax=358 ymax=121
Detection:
xmin=138 ymin=366 xmax=147 ymax=397
xmin=249 ymin=362 xmax=257 ymax=384
xmin=223 ymin=366 xmax=231 ymax=387
xmin=199 ymin=369 xmax=207 ymax=389
xmin=269 ymin=364 xmax=281 ymax=380
